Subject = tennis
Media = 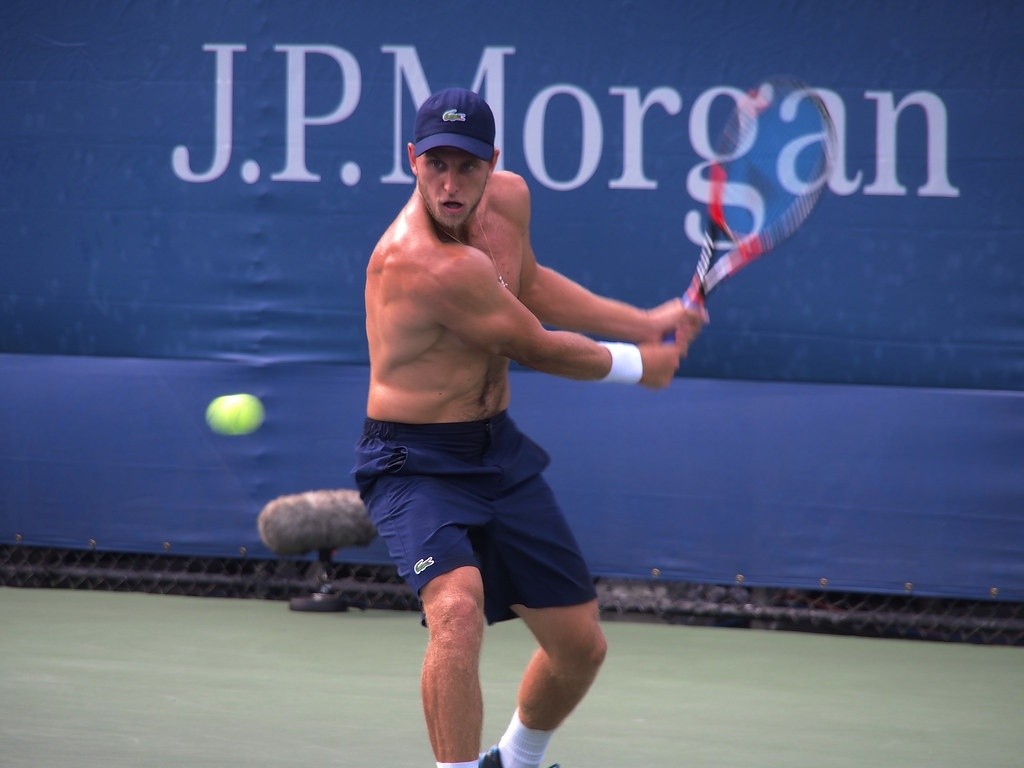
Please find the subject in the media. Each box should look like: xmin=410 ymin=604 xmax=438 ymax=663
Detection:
xmin=205 ymin=392 xmax=264 ymax=435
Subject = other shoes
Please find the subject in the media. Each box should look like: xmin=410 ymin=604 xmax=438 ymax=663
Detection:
xmin=480 ymin=744 xmax=504 ymax=768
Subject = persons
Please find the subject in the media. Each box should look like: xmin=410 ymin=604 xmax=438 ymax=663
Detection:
xmin=351 ymin=87 xmax=711 ymax=768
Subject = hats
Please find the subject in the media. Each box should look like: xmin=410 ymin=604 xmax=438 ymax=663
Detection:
xmin=413 ymin=87 xmax=497 ymax=164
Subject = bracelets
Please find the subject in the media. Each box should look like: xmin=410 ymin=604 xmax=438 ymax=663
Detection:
xmin=599 ymin=341 xmax=642 ymax=386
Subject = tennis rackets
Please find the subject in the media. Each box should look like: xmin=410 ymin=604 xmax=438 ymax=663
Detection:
xmin=660 ymin=76 xmax=836 ymax=354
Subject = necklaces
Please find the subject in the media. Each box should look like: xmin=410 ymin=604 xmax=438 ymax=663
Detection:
xmin=438 ymin=213 xmax=509 ymax=290
